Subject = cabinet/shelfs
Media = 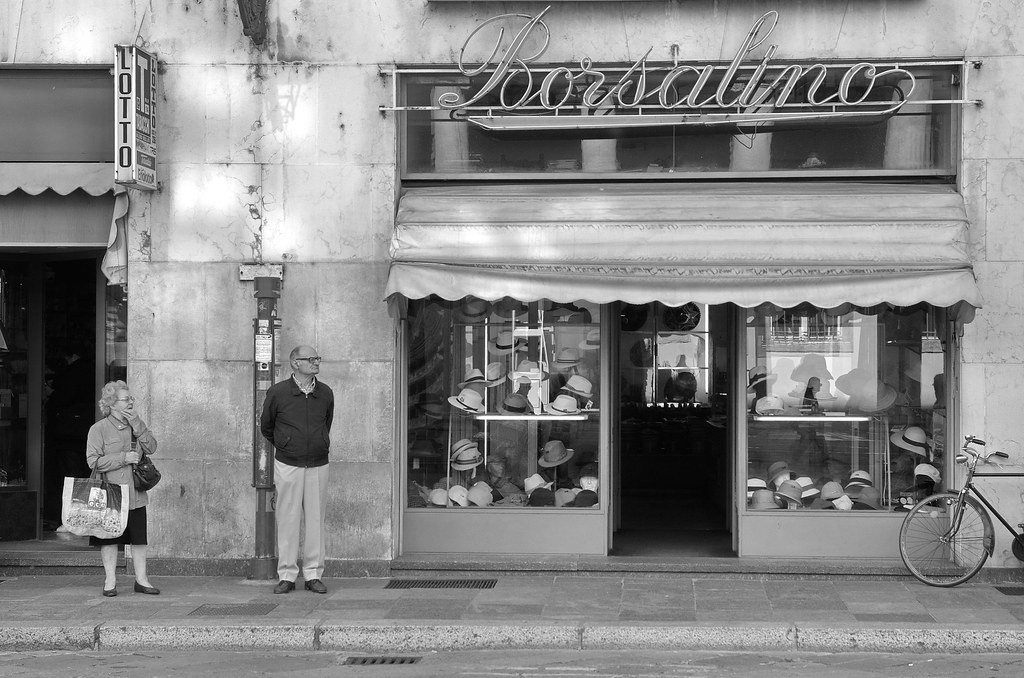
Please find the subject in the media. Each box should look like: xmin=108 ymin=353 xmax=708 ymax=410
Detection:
xmin=618 ymin=328 xmax=708 ymax=408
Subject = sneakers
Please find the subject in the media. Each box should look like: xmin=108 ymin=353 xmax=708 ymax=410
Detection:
xmin=305 ymin=579 xmax=327 ymax=593
xmin=274 ymin=580 xmax=295 ymax=594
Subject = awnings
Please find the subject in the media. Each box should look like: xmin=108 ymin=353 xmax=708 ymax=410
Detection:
xmin=384 ymin=184 xmax=980 ymax=309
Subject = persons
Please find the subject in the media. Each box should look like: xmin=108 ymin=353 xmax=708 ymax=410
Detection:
xmin=87 ymin=380 xmax=161 ymax=595
xmin=792 ymin=377 xmax=829 ymax=467
xmin=261 ymin=346 xmax=334 ymax=594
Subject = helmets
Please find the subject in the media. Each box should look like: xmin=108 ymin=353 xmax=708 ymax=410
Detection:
xmin=663 ymin=301 xmax=701 ymax=331
xmin=629 ymin=337 xmax=657 ymax=367
xmin=664 ymin=370 xmax=697 ymax=403
xmin=619 ymin=376 xmax=648 ymax=402
xmin=620 ymin=302 xmax=647 ymax=331
xmin=712 ymin=398 xmax=729 ymax=416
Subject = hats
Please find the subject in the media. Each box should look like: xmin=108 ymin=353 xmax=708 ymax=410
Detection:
xmin=743 ymin=296 xmax=949 ymax=515
xmin=410 ymin=297 xmax=599 ymax=509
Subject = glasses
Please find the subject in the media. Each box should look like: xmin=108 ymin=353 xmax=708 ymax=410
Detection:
xmin=297 ymin=356 xmax=321 ymax=364
xmin=116 ymin=395 xmax=135 ymax=404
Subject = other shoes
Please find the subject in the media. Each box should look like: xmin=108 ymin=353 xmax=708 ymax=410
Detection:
xmin=103 ymin=578 xmax=117 ymax=597
xmin=134 ymin=579 xmax=160 ymax=595
xmin=54 ymin=525 xmax=71 ymax=533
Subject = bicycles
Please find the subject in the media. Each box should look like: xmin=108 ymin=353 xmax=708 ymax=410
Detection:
xmin=898 ymin=434 xmax=1024 ymax=587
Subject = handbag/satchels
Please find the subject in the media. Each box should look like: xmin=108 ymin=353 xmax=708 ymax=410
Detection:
xmin=61 ymin=456 xmax=129 ymax=539
xmin=130 ymin=426 xmax=162 ymax=492
